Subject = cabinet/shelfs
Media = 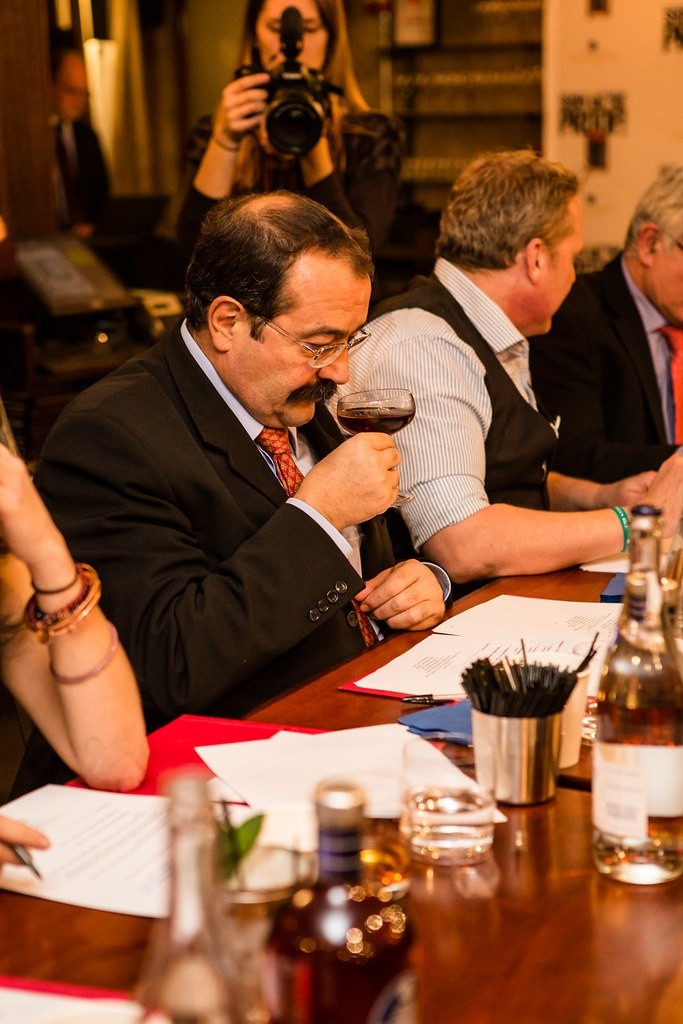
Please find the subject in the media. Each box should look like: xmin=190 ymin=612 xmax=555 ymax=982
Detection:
xmin=387 ymin=0 xmax=542 ymax=205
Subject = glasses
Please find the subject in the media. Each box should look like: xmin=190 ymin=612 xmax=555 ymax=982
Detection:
xmin=232 ymin=295 xmax=373 ymax=368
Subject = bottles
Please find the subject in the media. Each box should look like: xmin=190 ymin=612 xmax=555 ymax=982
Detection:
xmin=127 ymin=765 xmax=274 ymax=1024
xmin=263 ymin=778 xmax=422 ymax=1023
xmin=591 ymin=503 xmax=683 ymax=886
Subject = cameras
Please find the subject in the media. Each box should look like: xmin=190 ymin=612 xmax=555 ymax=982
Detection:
xmin=234 ymin=6 xmax=346 ymax=157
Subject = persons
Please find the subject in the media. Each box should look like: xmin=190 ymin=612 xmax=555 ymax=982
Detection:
xmin=323 ymin=151 xmax=683 ymax=604
xmin=170 ymin=0 xmax=399 ymax=247
xmin=0 ymin=399 xmax=149 ymax=875
xmin=25 ymin=190 xmax=455 ymax=792
xmin=49 ymin=43 xmax=112 ymax=238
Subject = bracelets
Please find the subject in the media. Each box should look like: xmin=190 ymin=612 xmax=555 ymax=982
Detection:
xmin=24 ymin=563 xmax=102 ymax=643
xmin=49 ymin=622 xmax=118 ymax=684
xmin=213 ymin=134 xmax=241 ymax=153
xmin=611 ymin=506 xmax=631 ymax=554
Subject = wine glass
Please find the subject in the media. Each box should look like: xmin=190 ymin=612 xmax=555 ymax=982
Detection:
xmin=337 ymin=387 xmax=416 ymax=509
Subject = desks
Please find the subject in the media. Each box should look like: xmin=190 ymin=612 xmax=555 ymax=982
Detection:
xmin=0 ymin=564 xmax=683 ymax=1023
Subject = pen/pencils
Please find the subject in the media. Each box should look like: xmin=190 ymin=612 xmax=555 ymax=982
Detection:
xmin=9 ymin=841 xmax=44 ymax=880
xmin=403 ymin=693 xmax=467 ymax=700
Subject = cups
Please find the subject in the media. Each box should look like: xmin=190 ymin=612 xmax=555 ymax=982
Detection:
xmin=469 ymin=706 xmax=562 ymax=804
xmin=196 ymin=845 xmax=318 ymax=1019
xmin=399 ymin=733 xmax=500 ymax=863
xmin=516 ymin=652 xmax=593 ymax=770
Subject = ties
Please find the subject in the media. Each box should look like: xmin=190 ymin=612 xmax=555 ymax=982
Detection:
xmin=657 ymin=325 xmax=683 ymax=447
xmin=254 ymin=424 xmax=377 ymax=651
